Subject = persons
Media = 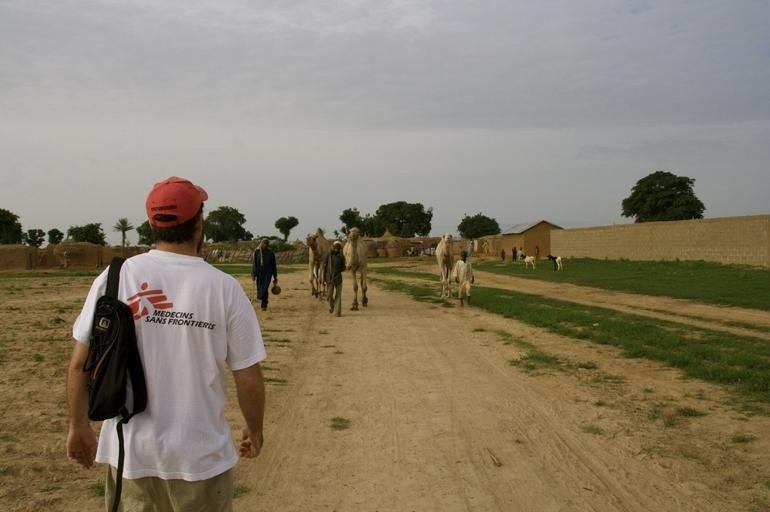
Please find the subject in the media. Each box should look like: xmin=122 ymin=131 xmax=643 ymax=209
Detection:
xmin=322 ymin=240 xmax=347 ymax=318
xmin=452 ymin=251 xmax=476 ymax=307
xmin=501 ymin=244 xmax=540 ymax=264
xmin=67 ymin=174 xmax=267 ymax=512
xmin=252 ymin=237 xmax=279 ymax=312
xmin=482 ymin=239 xmax=490 ymax=256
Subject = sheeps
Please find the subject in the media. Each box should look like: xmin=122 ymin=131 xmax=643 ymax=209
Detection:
xmin=548 ymin=254 xmax=562 ymax=272
xmin=520 ymin=253 xmax=537 ymax=269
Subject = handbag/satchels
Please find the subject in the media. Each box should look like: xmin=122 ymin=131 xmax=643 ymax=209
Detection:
xmin=82 ymin=255 xmax=148 ymax=420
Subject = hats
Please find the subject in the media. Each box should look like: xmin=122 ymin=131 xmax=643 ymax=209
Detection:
xmin=145 ymin=176 xmax=209 ymax=228
